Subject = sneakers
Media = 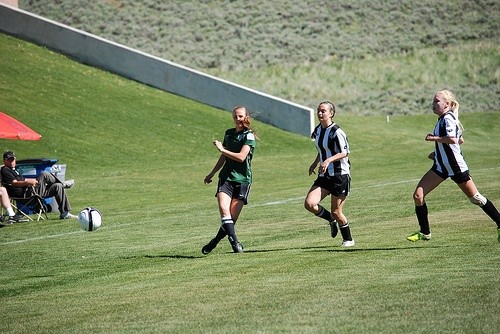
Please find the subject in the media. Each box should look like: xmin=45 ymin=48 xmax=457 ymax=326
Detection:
xmin=340 ymin=237 xmax=355 ymax=247
xmin=329 ymin=217 xmax=339 ymax=237
xmin=497 ymin=227 xmax=500 ymax=243
xmin=406 ymin=229 xmax=432 ymax=242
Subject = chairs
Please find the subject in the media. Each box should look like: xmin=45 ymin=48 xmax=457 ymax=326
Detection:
xmin=0 ymin=165 xmax=48 ymax=221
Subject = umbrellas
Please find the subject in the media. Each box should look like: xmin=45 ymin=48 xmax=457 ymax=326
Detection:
xmin=0 ymin=112 xmax=41 ymax=141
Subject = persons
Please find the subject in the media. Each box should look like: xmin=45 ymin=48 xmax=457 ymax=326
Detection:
xmin=303 ymin=100 xmax=356 ymax=247
xmin=0 ymin=186 xmax=30 ymax=226
xmin=406 ymin=89 xmax=500 ymax=246
xmin=201 ymin=105 xmax=256 ymax=256
xmin=0 ymin=151 xmax=80 ymax=219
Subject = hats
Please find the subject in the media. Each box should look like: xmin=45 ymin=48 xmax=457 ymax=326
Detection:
xmin=3 ymin=150 xmax=16 ymax=160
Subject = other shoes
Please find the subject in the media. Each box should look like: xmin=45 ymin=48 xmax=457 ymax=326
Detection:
xmin=202 ymin=237 xmax=217 ymax=254
xmin=232 ymin=240 xmax=243 ymax=252
xmin=62 ymin=178 xmax=74 ymax=188
xmin=0 ymin=214 xmax=4 ymax=222
xmin=8 ymin=215 xmax=21 ymax=223
xmin=63 ymin=212 xmax=78 ymax=219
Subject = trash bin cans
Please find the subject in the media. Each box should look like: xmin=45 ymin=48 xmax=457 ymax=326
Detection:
xmin=11 ymin=157 xmax=60 ymax=217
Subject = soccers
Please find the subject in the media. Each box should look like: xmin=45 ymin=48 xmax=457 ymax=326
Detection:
xmin=77 ymin=208 xmax=102 ymax=231
